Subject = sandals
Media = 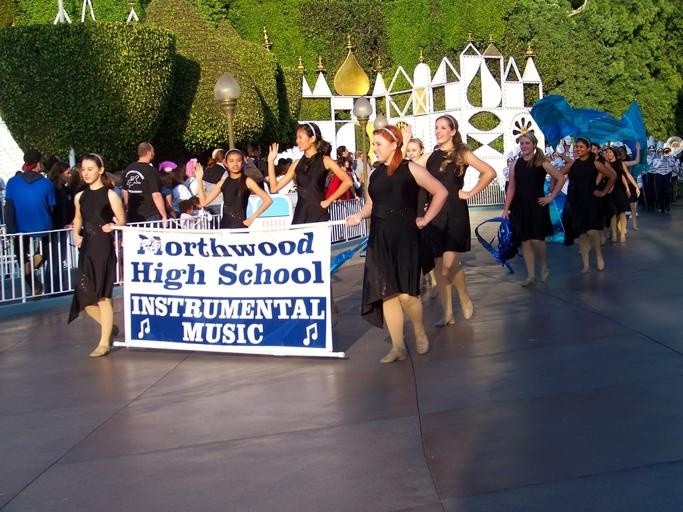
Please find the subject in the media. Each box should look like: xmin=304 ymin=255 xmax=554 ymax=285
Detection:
xmin=381 ymin=347 xmax=405 ymax=363
xmin=521 ymin=277 xmax=536 ymax=286
xmin=461 ymin=296 xmax=474 ymax=320
xmin=90 ymin=346 xmax=110 ymax=357
xmin=436 ymin=316 xmax=455 ymax=326
xmin=413 ymin=325 xmax=429 ymax=354
xmin=541 ymin=269 xmax=550 ymax=281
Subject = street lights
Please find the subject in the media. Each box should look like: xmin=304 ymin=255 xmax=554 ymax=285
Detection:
xmin=374 ymin=112 xmax=388 ymax=131
xmin=352 ymin=97 xmax=375 ymax=186
xmin=213 ymin=70 xmax=243 ymax=148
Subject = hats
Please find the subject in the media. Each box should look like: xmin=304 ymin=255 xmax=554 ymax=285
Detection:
xmin=21 ymin=150 xmax=41 ymax=171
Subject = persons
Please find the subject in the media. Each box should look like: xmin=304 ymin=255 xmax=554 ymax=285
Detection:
xmin=346 ymin=115 xmax=497 ymax=363
xmin=501 ymin=134 xmax=682 ymax=287
xmin=67 ymin=152 xmax=127 ymax=358
xmin=107 ymin=140 xmax=297 ymax=289
xmin=267 ymin=122 xmax=374 ymax=333
xmin=5 ymin=149 xmax=90 ymax=298
